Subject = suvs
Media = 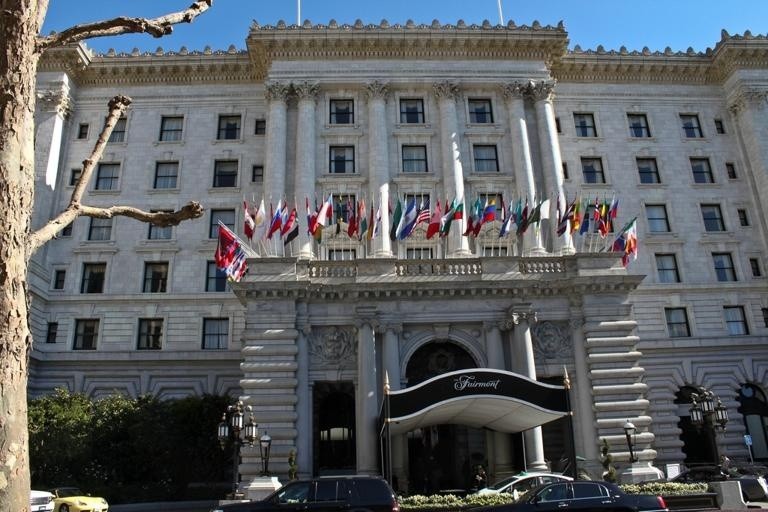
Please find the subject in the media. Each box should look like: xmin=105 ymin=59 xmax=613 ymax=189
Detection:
xmin=214 ymin=473 xmax=398 ymax=511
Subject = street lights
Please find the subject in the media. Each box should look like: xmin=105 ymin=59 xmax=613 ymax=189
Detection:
xmin=688 ymin=386 xmax=728 ymax=462
xmin=218 ymin=400 xmax=260 ymax=499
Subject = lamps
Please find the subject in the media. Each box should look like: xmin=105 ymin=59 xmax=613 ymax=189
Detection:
xmin=258 ymin=430 xmax=272 ymax=477
xmin=623 ymin=420 xmax=638 ymax=463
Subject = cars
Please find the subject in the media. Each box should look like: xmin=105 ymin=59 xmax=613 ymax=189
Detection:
xmin=635 ymin=465 xmax=768 ymax=502
xmin=456 ymin=473 xmax=669 ymax=512
xmin=31 ymin=486 xmax=110 ymax=512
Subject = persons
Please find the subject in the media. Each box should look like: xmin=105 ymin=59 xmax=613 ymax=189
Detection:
xmin=545 ymin=455 xmax=553 ymax=472
xmin=464 ymin=464 xmax=492 ymax=494
xmin=411 ymin=455 xmax=445 ymax=492
xmin=719 ymin=454 xmax=751 ymax=475
